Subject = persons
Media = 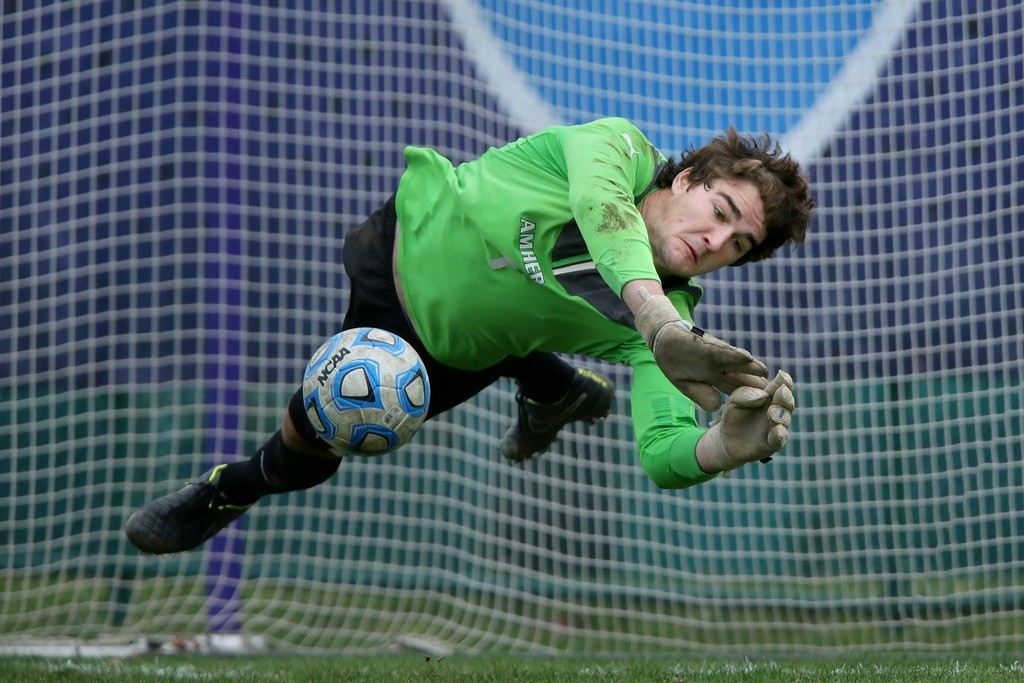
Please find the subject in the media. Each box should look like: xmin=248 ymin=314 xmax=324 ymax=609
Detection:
xmin=123 ymin=113 xmax=813 ymax=557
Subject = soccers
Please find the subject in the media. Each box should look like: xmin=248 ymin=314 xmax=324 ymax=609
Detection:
xmin=303 ymin=327 xmax=431 ymax=457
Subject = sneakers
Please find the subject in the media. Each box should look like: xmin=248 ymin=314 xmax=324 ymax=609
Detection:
xmin=122 ymin=462 xmax=262 ymax=554
xmin=499 ymin=367 xmax=612 ymax=460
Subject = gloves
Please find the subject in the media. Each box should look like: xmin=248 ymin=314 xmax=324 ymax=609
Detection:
xmin=636 ymin=298 xmax=766 ymax=413
xmin=702 ymin=369 xmax=792 ymax=471
